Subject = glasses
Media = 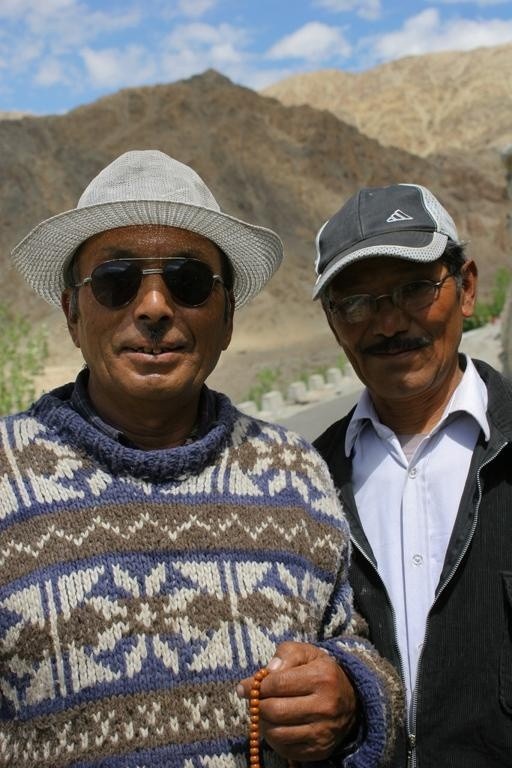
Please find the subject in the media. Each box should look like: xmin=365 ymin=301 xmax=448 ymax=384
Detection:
xmin=73 ymin=256 xmax=225 ymax=311
xmin=320 ymin=271 xmax=452 ymax=326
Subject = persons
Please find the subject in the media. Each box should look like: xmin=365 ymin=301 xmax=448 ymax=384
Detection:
xmin=0 ymin=150 xmax=406 ymax=768
xmin=304 ymin=182 xmax=512 ymax=767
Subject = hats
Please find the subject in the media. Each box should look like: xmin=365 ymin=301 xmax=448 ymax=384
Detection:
xmin=311 ymin=183 xmax=459 ymax=300
xmin=10 ymin=150 xmax=284 ymax=311
